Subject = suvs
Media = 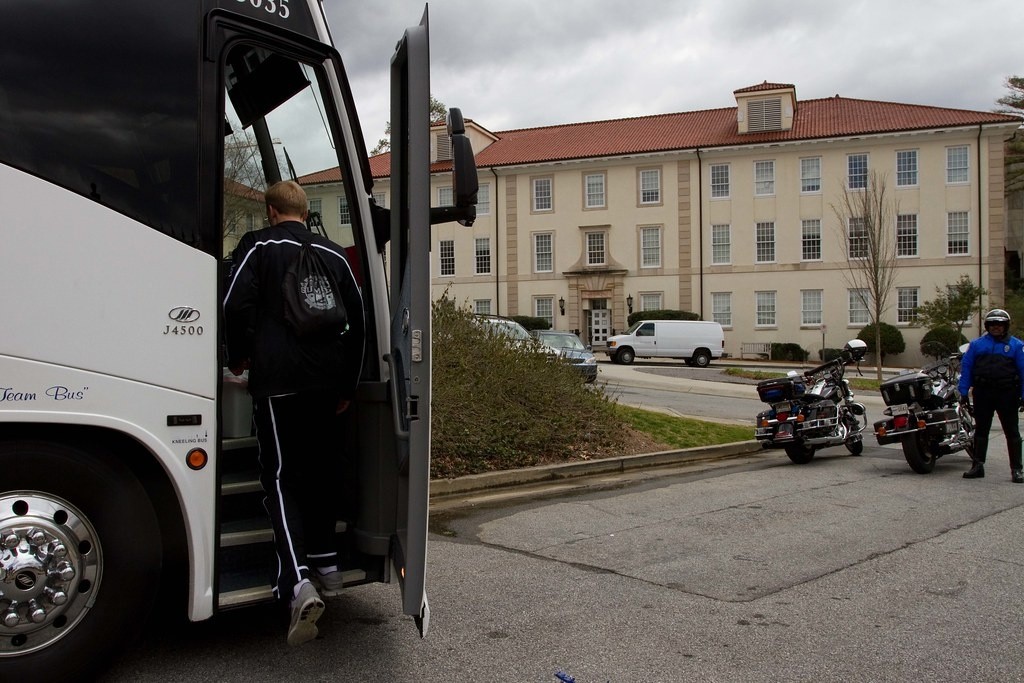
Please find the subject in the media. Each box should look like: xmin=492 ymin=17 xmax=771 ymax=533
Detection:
xmin=464 ymin=313 xmax=559 ymax=368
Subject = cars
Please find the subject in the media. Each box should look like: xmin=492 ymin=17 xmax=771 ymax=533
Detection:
xmin=529 ymin=330 xmax=598 ymax=383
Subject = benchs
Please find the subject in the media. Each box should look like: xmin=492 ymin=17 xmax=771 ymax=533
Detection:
xmin=740 ymin=341 xmax=772 ymax=362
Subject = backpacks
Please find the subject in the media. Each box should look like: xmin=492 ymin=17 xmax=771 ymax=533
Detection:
xmin=279 ymin=225 xmax=349 ymax=339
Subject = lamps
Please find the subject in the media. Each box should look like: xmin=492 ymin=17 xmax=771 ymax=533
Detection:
xmin=559 ymin=297 xmax=565 ymax=316
xmin=625 ymin=294 xmax=633 ymax=314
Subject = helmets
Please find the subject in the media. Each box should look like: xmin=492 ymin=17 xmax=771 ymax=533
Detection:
xmin=985 ymin=308 xmax=1011 ymax=331
xmin=844 ymin=339 xmax=867 ymax=360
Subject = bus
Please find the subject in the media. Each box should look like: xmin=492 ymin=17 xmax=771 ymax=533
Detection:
xmin=0 ymin=0 xmax=480 ymax=683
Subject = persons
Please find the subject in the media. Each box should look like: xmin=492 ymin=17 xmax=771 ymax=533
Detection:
xmin=957 ymin=309 xmax=1024 ymax=484
xmin=220 ymin=180 xmax=368 ymax=646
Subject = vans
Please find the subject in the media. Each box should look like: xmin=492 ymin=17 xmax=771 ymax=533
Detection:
xmin=605 ymin=320 xmax=725 ymax=368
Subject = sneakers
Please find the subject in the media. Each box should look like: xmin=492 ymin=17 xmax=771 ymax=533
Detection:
xmin=316 ymin=566 xmax=344 ymax=596
xmin=287 ymin=577 xmax=324 ymax=646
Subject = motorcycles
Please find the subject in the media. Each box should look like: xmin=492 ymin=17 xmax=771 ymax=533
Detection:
xmin=754 ymin=340 xmax=868 ymax=465
xmin=872 ymin=342 xmax=975 ymax=475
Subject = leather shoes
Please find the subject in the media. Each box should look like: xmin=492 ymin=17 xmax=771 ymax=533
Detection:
xmin=1012 ymin=468 xmax=1024 ymax=483
xmin=962 ymin=462 xmax=985 ymax=478
